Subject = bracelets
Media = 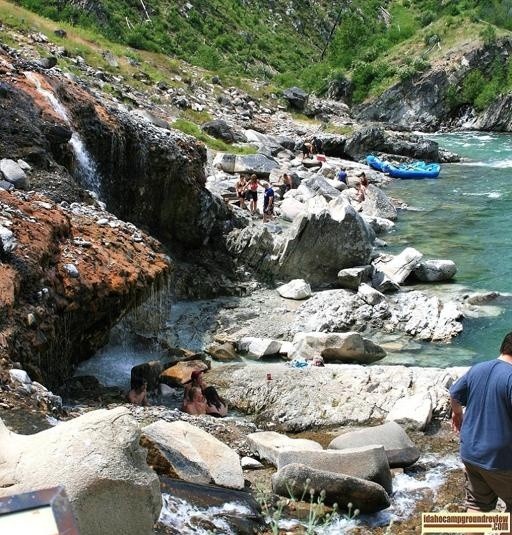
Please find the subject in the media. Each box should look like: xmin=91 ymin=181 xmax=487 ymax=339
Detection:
xmin=241 ymin=189 xmax=245 ymax=191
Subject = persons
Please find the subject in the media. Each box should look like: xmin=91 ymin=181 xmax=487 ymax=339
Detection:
xmin=282 ymin=172 xmax=293 ymax=194
xmin=358 ymin=171 xmax=368 ymax=187
xmin=182 ymin=369 xmax=209 ymax=412
xmin=262 ymin=182 xmax=276 ymax=224
xmin=125 ymin=377 xmax=150 ymax=406
xmin=235 ymin=174 xmax=249 ymax=210
xmin=335 ymin=167 xmax=349 ymax=185
xmin=302 ymin=142 xmax=317 ymax=159
xmin=201 ymin=386 xmax=230 ymax=418
xmin=354 ymin=182 xmax=366 ymax=203
xmin=184 ymin=388 xmax=209 ymax=416
xmin=374 ymin=152 xmax=410 ymax=169
xmin=449 ymin=332 xmax=510 ymax=514
xmin=241 ymin=173 xmax=263 ymax=213
xmin=312 ymin=136 xmax=324 ymax=154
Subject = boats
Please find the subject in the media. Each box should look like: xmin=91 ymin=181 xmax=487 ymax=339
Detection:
xmin=367 ymin=155 xmax=441 ymax=178
